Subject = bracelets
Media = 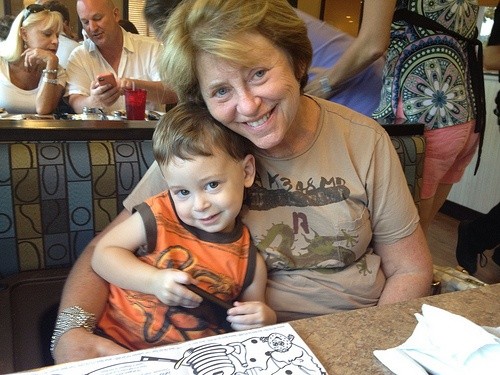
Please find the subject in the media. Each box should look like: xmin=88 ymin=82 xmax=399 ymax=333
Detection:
xmin=131 ymin=80 xmax=135 ymax=91
xmin=43 ymin=69 xmax=57 ymax=73
xmin=50 ymin=306 xmax=97 ymax=359
xmin=42 ymin=77 xmax=56 ymax=84
xmin=320 ymin=74 xmax=341 ymax=94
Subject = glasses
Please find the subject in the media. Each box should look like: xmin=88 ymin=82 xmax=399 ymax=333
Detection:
xmin=21 ymin=1 xmax=59 ymax=26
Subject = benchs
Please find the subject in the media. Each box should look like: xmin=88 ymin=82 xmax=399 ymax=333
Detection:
xmin=1 ymin=115 xmax=426 ymax=374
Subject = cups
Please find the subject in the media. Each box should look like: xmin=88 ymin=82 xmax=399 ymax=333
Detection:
xmin=125 ymin=89 xmax=146 ymax=121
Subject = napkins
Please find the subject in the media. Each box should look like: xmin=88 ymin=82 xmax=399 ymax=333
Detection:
xmin=374 ymin=301 xmax=500 ymax=375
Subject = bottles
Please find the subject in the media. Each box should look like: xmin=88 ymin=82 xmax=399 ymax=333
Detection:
xmin=84 ymin=107 xmax=96 ymax=120
xmin=114 ymin=111 xmax=122 ymax=120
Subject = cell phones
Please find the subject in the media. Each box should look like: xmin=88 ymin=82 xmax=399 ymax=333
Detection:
xmin=178 ymin=284 xmax=240 ymax=330
xmin=97 ymin=72 xmax=118 ymax=96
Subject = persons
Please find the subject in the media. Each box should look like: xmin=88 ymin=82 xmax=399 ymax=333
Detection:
xmin=302 ymin=0 xmax=480 ymax=229
xmin=0 ymin=0 xmax=139 ymax=69
xmin=59 ymin=0 xmax=177 ymax=113
xmin=50 ymin=0 xmax=432 ymax=363
xmin=144 ymin=0 xmax=383 ymax=116
xmin=85 ymin=100 xmax=277 ymax=352
xmin=0 ymin=4 xmax=67 ymax=114
xmin=456 ymin=2 xmax=500 ymax=273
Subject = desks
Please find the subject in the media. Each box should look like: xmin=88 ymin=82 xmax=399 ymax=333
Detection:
xmin=9 ymin=281 xmax=500 ymax=375
